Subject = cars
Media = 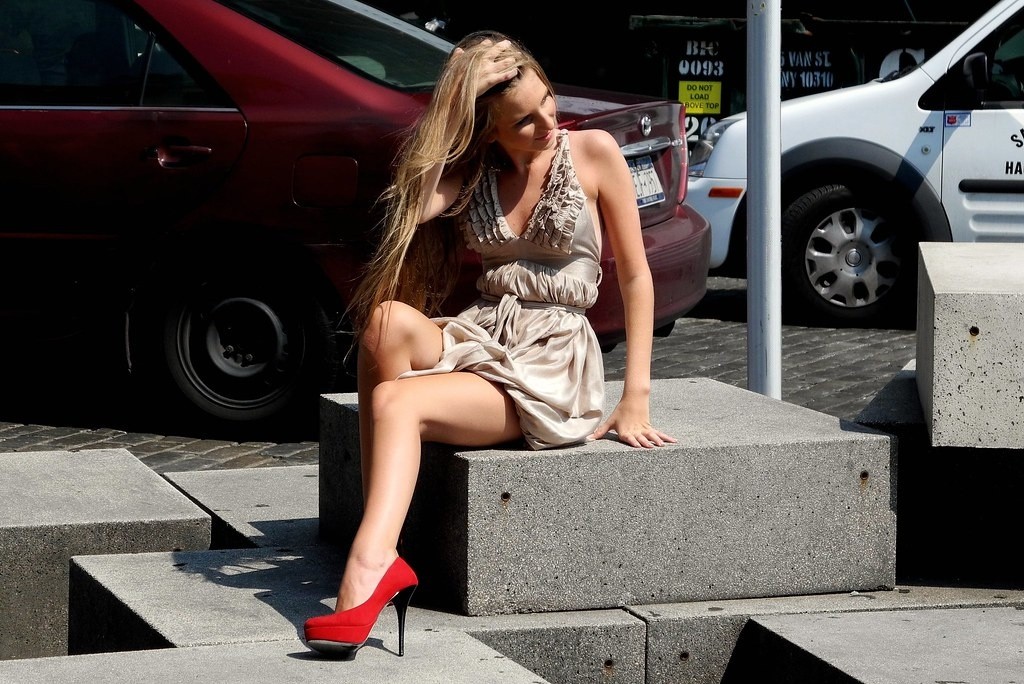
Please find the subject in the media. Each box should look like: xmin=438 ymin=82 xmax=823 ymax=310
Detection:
xmin=0 ymin=0 xmax=709 ymax=437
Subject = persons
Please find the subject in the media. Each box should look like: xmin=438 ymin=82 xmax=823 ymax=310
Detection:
xmin=302 ymin=32 xmax=681 ymax=662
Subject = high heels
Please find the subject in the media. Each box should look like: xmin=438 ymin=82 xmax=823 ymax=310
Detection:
xmin=304 ymin=557 xmax=418 ymax=661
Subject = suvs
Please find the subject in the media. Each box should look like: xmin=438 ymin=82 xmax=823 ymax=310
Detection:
xmin=683 ymin=0 xmax=1024 ymax=330
